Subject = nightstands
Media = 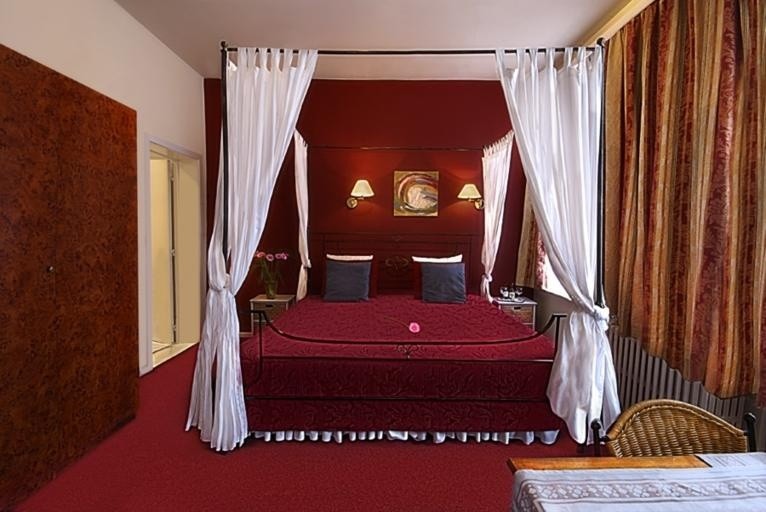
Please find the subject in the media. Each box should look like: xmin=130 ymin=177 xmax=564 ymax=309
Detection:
xmin=250 ymin=293 xmax=294 ymax=334
xmin=495 ymin=297 xmax=537 ymax=330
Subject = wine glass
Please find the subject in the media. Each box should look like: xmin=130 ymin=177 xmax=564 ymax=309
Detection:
xmin=499 ymin=286 xmax=523 ymax=301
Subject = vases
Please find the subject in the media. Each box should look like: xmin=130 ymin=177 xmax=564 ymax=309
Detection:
xmin=262 ymin=279 xmax=278 ymax=298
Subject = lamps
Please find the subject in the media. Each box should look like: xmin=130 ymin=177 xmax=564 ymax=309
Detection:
xmin=456 ymin=183 xmax=485 ymax=212
xmin=344 ymin=178 xmax=374 ymax=209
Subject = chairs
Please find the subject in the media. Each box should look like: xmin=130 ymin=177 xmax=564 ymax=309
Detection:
xmin=589 ymin=396 xmax=760 ymax=457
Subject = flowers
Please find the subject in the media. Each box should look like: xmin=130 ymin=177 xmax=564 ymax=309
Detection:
xmin=251 ymin=248 xmax=289 ymax=294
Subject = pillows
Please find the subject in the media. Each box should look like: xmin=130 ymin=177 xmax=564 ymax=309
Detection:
xmin=323 ymin=261 xmax=371 ymax=302
xmin=412 ymin=253 xmax=464 ymax=265
xmin=409 ymin=261 xmax=422 ymax=298
xmin=421 ymin=260 xmax=467 ymax=304
xmin=326 ymin=252 xmax=373 ymax=262
xmin=320 ymin=257 xmax=379 ymax=299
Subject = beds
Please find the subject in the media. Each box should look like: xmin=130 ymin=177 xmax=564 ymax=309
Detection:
xmin=189 ymin=36 xmax=615 ymax=446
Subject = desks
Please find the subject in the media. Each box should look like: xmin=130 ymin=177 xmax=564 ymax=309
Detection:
xmin=490 ymin=451 xmax=766 ymax=512
